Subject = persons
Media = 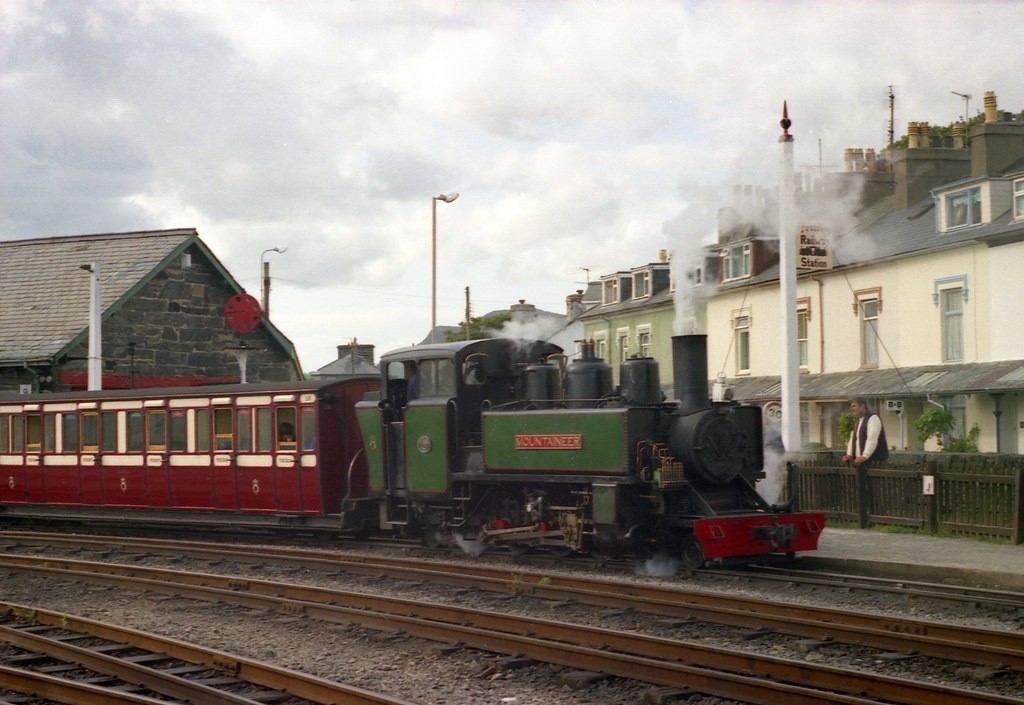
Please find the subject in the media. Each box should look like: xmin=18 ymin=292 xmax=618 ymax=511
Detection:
xmin=838 ymin=395 xmax=890 ymax=514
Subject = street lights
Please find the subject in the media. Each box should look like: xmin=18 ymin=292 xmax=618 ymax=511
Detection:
xmin=431 ymin=191 xmax=459 ymax=343
xmin=260 ymin=245 xmax=288 ymax=314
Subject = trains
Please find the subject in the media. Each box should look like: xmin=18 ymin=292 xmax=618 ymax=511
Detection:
xmin=1 ymin=337 xmax=832 ymax=582
